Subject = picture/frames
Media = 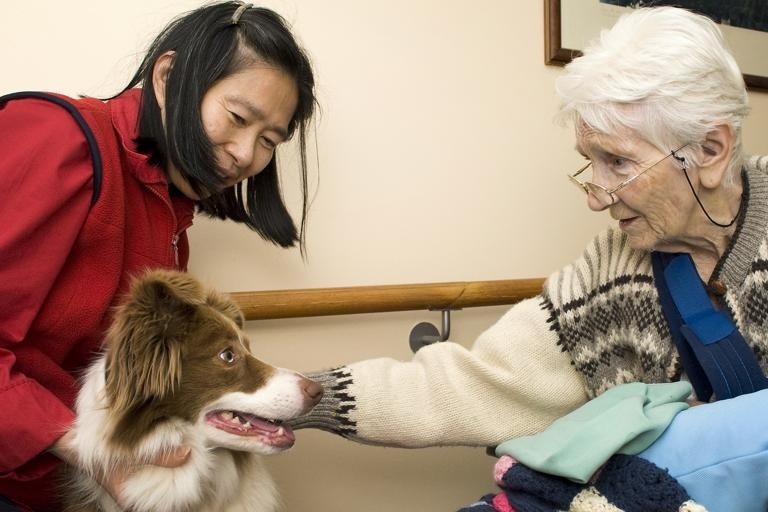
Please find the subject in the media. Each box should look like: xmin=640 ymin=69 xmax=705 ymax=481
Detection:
xmin=544 ymin=0 xmax=768 ymax=89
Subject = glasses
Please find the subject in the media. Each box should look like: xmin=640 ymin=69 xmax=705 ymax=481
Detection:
xmin=566 ymin=142 xmax=717 ymax=207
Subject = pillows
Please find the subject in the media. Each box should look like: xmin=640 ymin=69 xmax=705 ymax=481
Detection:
xmin=638 ymin=387 xmax=768 ymax=512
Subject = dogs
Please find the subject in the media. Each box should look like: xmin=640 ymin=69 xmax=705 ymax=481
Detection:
xmin=46 ymin=264 xmax=325 ymax=512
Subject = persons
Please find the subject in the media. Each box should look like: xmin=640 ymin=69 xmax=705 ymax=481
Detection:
xmin=1 ymin=2 xmax=325 ymax=512
xmin=271 ymin=6 xmax=766 ymax=512
